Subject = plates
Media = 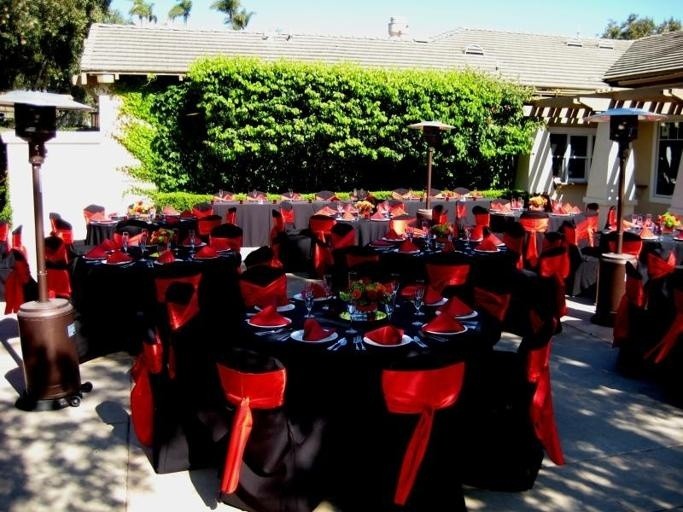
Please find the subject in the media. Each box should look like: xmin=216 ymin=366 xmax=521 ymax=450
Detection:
xmin=245 ymin=315 xmax=293 ymax=328
xmin=82 ymin=228 xmax=221 ymax=266
xmin=254 ymin=304 xmax=296 ymax=313
xmin=429 ymin=297 xmax=448 ymax=306
xmin=488 ymin=207 xmax=582 ymax=216
xmin=332 ymin=207 xmax=393 ymax=222
xmin=290 ymin=328 xmax=338 ymax=343
xmin=422 ymin=308 xmax=480 ymax=336
xmin=361 ymin=330 xmax=412 ymax=347
xmin=292 ymin=292 xmax=330 ymax=302
xmin=368 ymin=234 xmax=507 ymax=254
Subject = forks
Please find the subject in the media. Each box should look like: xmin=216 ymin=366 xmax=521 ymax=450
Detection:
xmin=352 ymin=334 xmax=367 ymax=352
xmin=413 ymin=330 xmax=450 ymax=348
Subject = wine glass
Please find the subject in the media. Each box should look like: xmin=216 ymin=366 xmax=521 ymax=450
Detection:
xmin=403 ymin=219 xmax=474 ymax=252
xmin=630 ymin=212 xmax=652 ymax=231
xmin=304 ymin=270 xmax=428 ymax=328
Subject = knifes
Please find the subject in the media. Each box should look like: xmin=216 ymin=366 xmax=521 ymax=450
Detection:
xmin=328 ymin=336 xmax=345 ymax=350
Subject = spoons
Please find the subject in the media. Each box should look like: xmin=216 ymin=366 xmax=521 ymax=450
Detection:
xmin=254 ymin=328 xmax=296 ymax=342
xmin=332 ymin=339 xmax=348 ymax=351
